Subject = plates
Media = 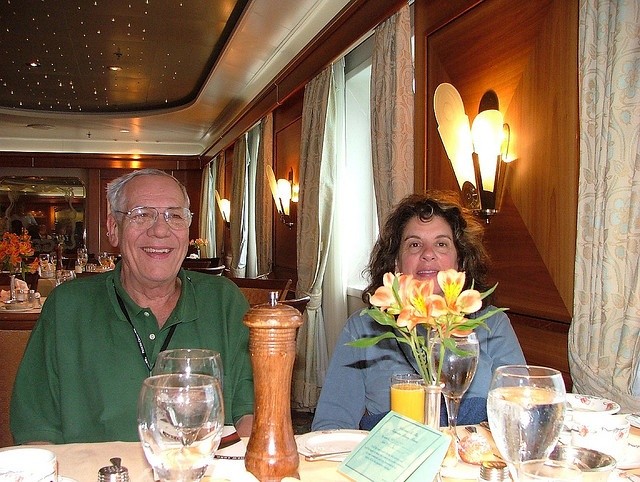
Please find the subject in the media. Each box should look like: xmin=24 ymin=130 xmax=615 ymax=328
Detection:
xmin=294 ymin=427 xmax=373 ymax=463
xmin=564 ymin=391 xmax=621 ymax=416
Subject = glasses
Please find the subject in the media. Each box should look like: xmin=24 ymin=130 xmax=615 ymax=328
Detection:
xmin=114 ymin=206 xmax=194 ymax=231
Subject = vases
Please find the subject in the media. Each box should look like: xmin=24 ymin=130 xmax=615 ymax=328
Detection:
xmin=425 ymin=387 xmax=442 ymax=431
xmin=198 ymin=249 xmax=200 ymax=259
xmin=6 ymin=275 xmax=23 ymax=303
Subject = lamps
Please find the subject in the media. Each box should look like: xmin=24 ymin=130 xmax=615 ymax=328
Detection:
xmin=214 ymin=190 xmax=231 ymax=228
xmin=433 ymin=81 xmax=511 ymax=226
xmin=265 ymin=163 xmax=299 ymax=231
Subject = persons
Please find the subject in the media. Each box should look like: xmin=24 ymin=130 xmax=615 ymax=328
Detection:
xmin=311 ymin=191 xmax=531 ymax=430
xmin=9 ymin=170 xmax=262 ymax=444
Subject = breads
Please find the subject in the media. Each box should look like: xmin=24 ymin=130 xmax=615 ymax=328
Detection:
xmin=458 ymin=432 xmax=499 ymax=465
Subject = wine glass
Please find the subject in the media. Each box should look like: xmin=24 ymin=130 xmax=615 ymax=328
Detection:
xmin=428 ymin=329 xmax=480 ymax=441
xmin=487 ymin=364 xmax=568 ymax=480
xmin=514 ymin=444 xmax=617 ymax=480
xmin=153 ymin=347 xmax=225 ymax=451
xmin=136 ymin=372 xmax=225 ymax=481
xmin=77 ymin=249 xmax=88 ymax=266
xmin=96 ymin=251 xmax=106 ymax=265
xmin=55 ymin=269 xmax=77 ymax=286
xmin=38 ymin=252 xmax=49 ymax=266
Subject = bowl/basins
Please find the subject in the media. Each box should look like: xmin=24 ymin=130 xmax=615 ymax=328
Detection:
xmin=570 ymin=416 xmax=631 ymax=448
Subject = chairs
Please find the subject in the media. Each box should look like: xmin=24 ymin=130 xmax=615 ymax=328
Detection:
xmin=189 ymin=264 xmax=225 ymax=276
xmin=250 ymin=297 xmax=311 ymax=343
xmin=228 ymin=276 xmax=293 ymax=309
xmin=1 ymin=309 xmax=43 ymax=448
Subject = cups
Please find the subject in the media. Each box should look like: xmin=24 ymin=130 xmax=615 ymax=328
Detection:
xmin=389 ymin=371 xmax=426 ymax=424
xmin=22 ymin=289 xmax=36 ymax=310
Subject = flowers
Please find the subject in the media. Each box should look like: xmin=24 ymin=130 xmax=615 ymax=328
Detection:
xmin=0 ymin=229 xmax=36 ymax=275
xmin=339 ymin=267 xmax=512 ymax=382
xmin=190 ymin=238 xmax=208 ymax=249
